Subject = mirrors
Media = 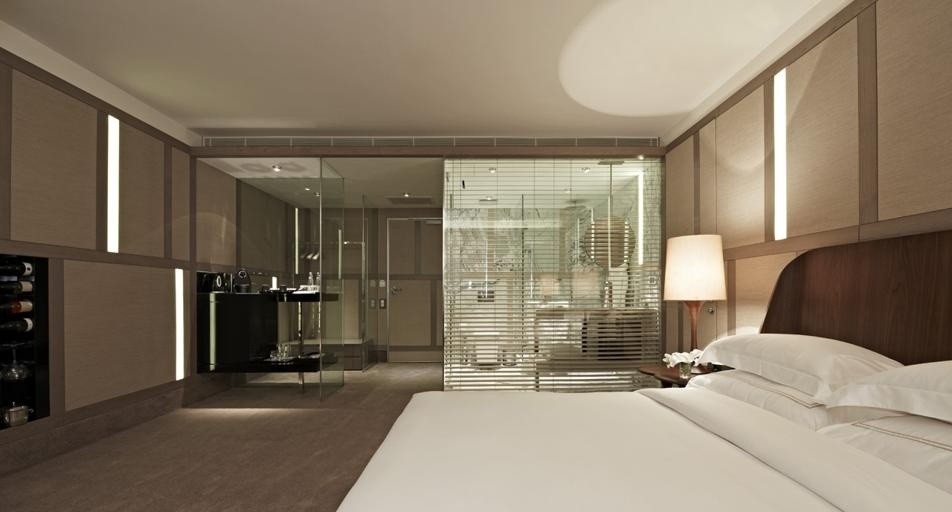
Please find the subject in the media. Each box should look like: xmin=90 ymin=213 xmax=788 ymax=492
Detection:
xmin=584 ymin=215 xmax=635 ymax=270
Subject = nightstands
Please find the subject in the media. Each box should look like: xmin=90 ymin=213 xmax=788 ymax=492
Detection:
xmin=637 ymin=366 xmax=720 ymax=387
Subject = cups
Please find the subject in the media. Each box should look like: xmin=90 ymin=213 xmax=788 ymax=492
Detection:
xmin=280 ymin=285 xmax=286 ymax=292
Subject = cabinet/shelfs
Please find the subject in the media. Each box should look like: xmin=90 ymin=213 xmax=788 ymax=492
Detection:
xmin=196 ymin=293 xmax=339 ymax=395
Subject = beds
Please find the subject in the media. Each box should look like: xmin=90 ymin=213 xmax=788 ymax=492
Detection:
xmin=337 ymin=231 xmax=952 ymax=510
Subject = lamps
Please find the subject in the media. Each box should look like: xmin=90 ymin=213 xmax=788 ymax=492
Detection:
xmin=664 ymin=235 xmax=728 ymax=373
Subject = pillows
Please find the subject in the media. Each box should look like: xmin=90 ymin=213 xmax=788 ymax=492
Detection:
xmin=693 ymin=334 xmax=951 ymax=424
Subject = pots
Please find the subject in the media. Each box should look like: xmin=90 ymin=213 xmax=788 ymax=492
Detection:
xmin=1 ymin=400 xmax=36 ymax=428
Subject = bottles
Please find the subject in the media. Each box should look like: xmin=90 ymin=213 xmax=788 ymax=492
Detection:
xmin=1 ymin=298 xmax=34 ymax=315
xmin=1 ymin=261 xmax=35 ymax=277
xmin=1 ymin=317 xmax=34 ymax=335
xmin=316 ymin=272 xmax=320 ymax=285
xmin=308 ymin=272 xmax=314 ymax=285
xmin=0 ymin=278 xmax=34 ymax=296
